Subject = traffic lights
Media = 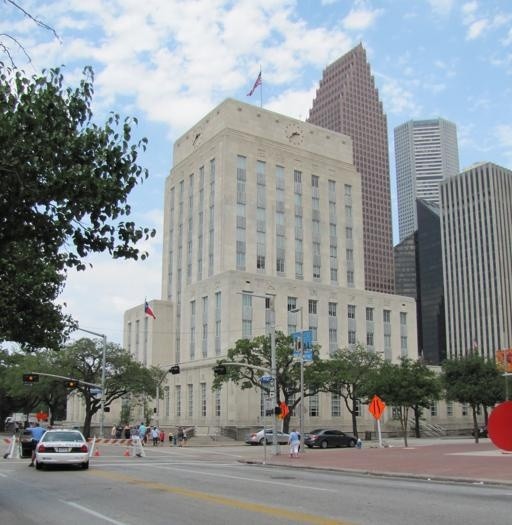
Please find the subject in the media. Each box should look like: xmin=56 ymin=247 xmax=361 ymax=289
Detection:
xmin=64 ymin=381 xmax=81 ymax=388
xmin=22 ymin=374 xmax=39 ymax=382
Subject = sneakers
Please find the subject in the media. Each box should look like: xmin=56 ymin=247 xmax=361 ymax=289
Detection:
xmin=28 ymin=463 xmax=33 ymax=466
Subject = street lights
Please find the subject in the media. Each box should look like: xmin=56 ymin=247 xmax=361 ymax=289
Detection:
xmin=290 ymin=306 xmax=306 ymax=454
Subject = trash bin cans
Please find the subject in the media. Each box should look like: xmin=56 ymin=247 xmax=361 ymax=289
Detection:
xmin=366 ymin=431 xmax=371 ymax=440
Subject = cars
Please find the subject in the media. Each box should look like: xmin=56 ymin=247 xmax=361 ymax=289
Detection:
xmin=246 ymin=429 xmax=290 ymax=446
xmin=470 ymin=426 xmax=489 ymax=438
xmin=18 ymin=426 xmax=89 ymax=470
xmin=304 ymin=429 xmax=358 ymax=449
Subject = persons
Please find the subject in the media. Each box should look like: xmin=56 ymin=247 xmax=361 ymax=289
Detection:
xmin=287 ymin=430 xmax=301 ymax=459
xmin=25 ymin=422 xmax=46 ymax=467
xmin=111 ymin=421 xmax=189 ymax=447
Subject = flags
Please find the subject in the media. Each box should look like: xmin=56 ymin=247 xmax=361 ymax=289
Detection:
xmin=145 ymin=301 xmax=157 ymax=320
xmin=246 ymin=70 xmax=262 ymax=97
